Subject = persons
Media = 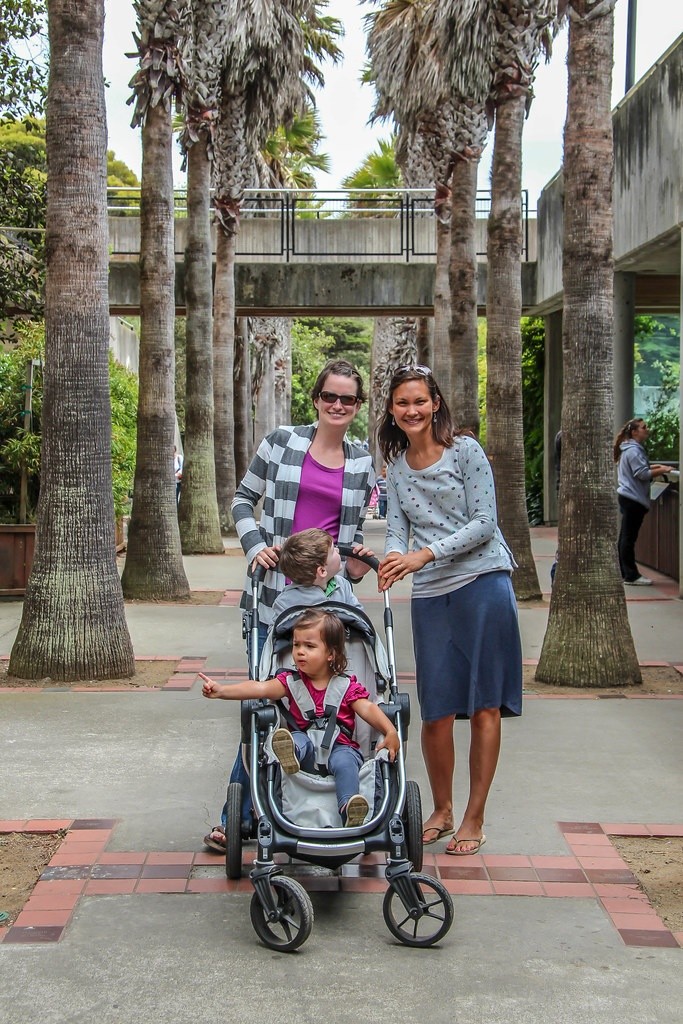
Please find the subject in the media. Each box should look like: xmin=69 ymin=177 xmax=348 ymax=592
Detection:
xmin=376 ymin=466 xmax=388 ymax=519
xmin=199 ymin=609 xmax=399 ymax=829
xmin=612 ymin=418 xmax=673 ymax=585
xmin=378 ymin=366 xmax=523 ymax=855
xmin=268 ymin=528 xmax=362 ymax=632
xmin=205 ymin=360 xmax=377 ymax=854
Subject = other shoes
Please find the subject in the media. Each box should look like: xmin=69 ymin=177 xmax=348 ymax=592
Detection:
xmin=380 ymin=515 xmax=386 ymax=519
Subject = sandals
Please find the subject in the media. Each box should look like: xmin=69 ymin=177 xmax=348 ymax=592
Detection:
xmin=204 ymin=825 xmax=227 ymax=854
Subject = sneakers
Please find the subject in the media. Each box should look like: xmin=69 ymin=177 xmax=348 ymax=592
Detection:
xmin=624 ymin=576 xmax=653 ymax=586
xmin=340 ymin=794 xmax=369 ymax=828
xmin=272 ymin=728 xmax=300 ymax=775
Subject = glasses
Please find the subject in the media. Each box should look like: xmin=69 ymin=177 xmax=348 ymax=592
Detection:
xmin=394 ymin=365 xmax=432 ymax=377
xmin=316 ymin=391 xmax=360 ymax=407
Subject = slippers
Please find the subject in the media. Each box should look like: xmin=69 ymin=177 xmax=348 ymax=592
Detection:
xmin=421 ymin=823 xmax=455 ymax=845
xmin=445 ymin=833 xmax=487 ymax=855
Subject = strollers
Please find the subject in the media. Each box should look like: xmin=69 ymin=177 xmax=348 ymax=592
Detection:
xmin=222 ymin=544 xmax=457 ymax=957
